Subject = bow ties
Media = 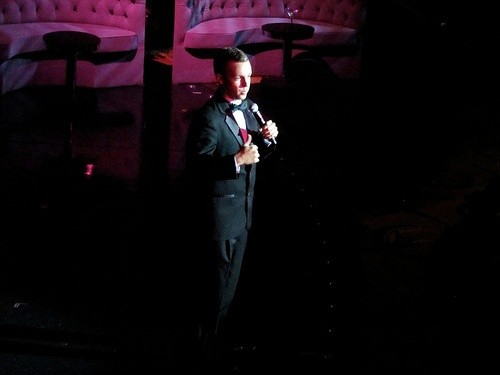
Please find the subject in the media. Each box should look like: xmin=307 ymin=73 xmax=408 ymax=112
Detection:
xmin=230 ymin=100 xmax=247 ymax=113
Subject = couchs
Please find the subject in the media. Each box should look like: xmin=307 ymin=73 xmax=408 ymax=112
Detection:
xmin=171 ymin=1 xmax=370 ymax=85
xmin=0 ymin=0 xmax=141 ymax=88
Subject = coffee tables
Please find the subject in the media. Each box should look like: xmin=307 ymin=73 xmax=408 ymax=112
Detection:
xmin=43 ymin=30 xmax=102 ymax=141
xmin=260 ymin=21 xmax=315 ymax=89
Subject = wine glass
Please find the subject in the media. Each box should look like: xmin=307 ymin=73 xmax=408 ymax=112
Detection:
xmin=287 ymin=4 xmax=299 ymax=23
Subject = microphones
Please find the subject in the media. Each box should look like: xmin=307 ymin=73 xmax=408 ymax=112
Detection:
xmin=250 ymin=103 xmax=278 ymax=145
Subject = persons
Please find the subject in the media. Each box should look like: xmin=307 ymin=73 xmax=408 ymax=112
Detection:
xmin=177 ymin=44 xmax=281 ymax=337
